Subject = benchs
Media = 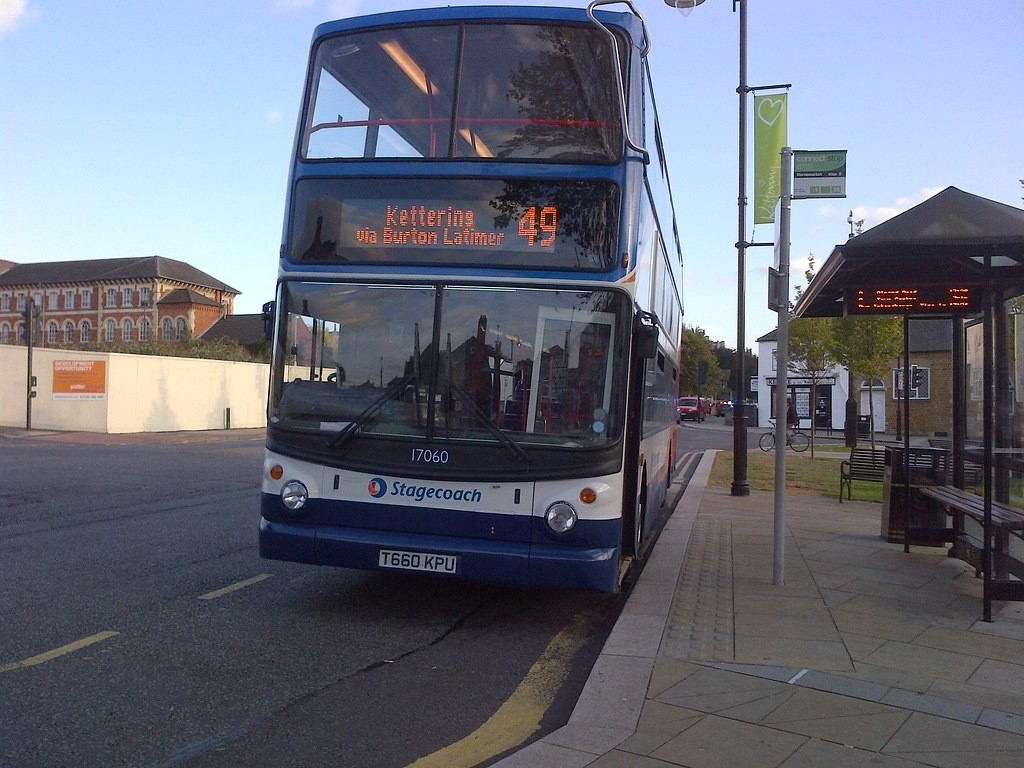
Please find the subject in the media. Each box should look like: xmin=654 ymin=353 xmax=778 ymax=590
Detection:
xmin=903 ymin=483 xmax=1024 ymax=623
xmin=838 ymin=446 xmax=982 ymax=503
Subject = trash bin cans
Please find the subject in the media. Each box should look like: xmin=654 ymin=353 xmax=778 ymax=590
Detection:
xmin=856 ymin=415 xmax=870 ymax=438
xmin=881 ymin=443 xmax=950 ymax=547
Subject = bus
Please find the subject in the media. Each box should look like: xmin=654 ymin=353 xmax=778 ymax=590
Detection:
xmin=257 ymin=0 xmax=688 ymax=599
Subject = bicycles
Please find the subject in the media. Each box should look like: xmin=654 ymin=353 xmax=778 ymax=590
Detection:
xmin=759 ymin=419 xmax=810 ymax=453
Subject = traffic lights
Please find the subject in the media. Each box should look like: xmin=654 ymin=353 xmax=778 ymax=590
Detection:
xmin=910 ymin=364 xmax=922 ymax=391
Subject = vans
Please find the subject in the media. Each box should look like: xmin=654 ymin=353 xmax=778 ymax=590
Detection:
xmin=676 ymin=396 xmax=707 ymax=422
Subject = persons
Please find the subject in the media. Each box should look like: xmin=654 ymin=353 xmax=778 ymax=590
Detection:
xmin=786 ymin=398 xmax=798 ymax=445
